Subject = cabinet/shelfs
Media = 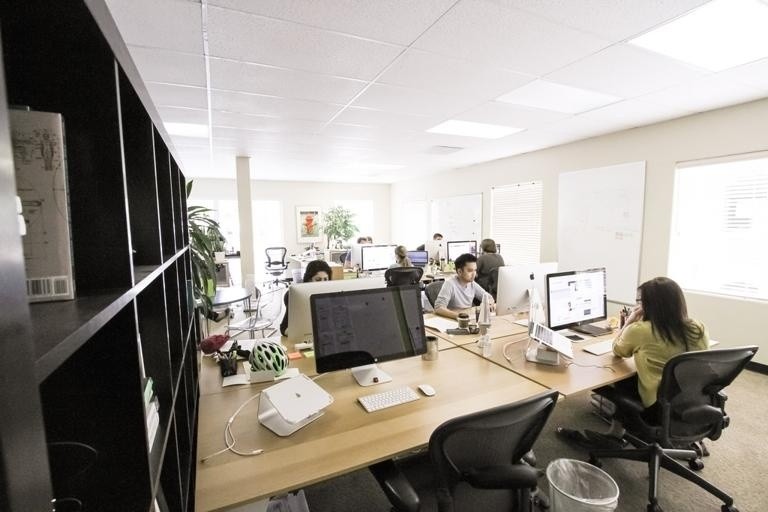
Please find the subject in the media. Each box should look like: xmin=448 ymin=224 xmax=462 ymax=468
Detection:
xmin=0 ymin=0 xmax=203 ymax=512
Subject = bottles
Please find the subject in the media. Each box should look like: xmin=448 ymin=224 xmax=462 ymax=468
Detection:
xmin=441 ymin=258 xmax=445 ymax=271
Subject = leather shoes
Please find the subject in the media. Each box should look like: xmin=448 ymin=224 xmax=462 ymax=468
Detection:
xmin=584 ymin=429 xmax=624 ymax=449
xmin=215 ymin=308 xmax=230 ymax=322
xmin=558 ymin=427 xmax=592 ymax=448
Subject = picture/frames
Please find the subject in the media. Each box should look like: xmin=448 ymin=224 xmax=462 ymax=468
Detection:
xmin=296 ymin=207 xmax=322 ymax=244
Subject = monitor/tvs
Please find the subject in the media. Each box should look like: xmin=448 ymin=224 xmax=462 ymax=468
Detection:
xmin=310 ymin=284 xmax=428 ymax=384
xmin=424 ymin=239 xmax=446 ymax=267
xmin=352 ymin=244 xmax=368 ymax=270
xmin=445 ymin=240 xmax=479 ymax=265
xmin=547 ymin=267 xmax=615 ymax=336
xmin=480 ymin=245 xmax=500 ymax=254
xmin=495 ymin=263 xmax=559 ymax=328
xmin=361 ymin=244 xmax=398 ymax=277
xmin=408 ymin=251 xmax=428 ymax=267
xmin=287 ymin=278 xmax=391 ymax=358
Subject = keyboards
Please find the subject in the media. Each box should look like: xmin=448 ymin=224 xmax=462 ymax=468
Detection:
xmin=583 ymin=338 xmax=614 ymax=356
xmin=357 ymin=386 xmax=420 ymax=414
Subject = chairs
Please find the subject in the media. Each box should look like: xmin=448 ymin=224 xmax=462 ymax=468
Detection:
xmin=368 ymin=389 xmax=559 ymax=512
xmin=263 ymin=247 xmax=291 ymax=289
xmin=223 ymin=287 xmax=284 ymax=340
xmin=589 ymin=345 xmax=768 ymax=512
xmin=384 ymin=267 xmax=423 ymax=286
xmin=425 ymin=281 xmax=445 ymax=309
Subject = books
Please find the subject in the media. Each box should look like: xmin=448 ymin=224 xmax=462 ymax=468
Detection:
xmin=5 ymin=109 xmax=78 ymax=303
xmin=151 ymin=481 xmax=171 ymax=512
xmin=138 ymin=333 xmax=161 ymax=455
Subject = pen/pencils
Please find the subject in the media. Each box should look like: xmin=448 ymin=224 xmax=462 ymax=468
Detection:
xmin=217 ymin=349 xmax=236 ymax=377
xmin=619 ymin=306 xmax=631 ymax=317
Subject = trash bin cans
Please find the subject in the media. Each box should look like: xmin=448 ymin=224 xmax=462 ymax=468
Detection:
xmin=546 ymin=458 xmax=620 ymax=512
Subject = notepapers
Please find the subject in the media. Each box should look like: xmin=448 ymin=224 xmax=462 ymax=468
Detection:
xmin=304 ymin=351 xmax=315 ymax=358
xmin=288 ymin=352 xmax=302 ymax=359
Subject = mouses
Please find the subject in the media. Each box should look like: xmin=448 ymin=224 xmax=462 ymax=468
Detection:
xmin=417 ymin=384 xmax=436 ymax=396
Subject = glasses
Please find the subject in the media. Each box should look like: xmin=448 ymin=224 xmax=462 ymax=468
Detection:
xmin=636 ymin=294 xmax=641 ymax=304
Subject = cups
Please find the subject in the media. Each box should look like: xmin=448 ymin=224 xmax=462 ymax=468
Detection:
xmin=421 ymin=336 xmax=438 ymax=360
xmin=458 ymin=313 xmax=470 ymax=329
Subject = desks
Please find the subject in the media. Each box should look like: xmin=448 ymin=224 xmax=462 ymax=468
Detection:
xmin=422 ymin=305 xmax=530 ymax=346
xmin=197 ymin=328 xmax=459 ymax=396
xmin=212 ymin=250 xmax=241 ymax=287
xmin=194 ymin=346 xmax=566 ymax=512
xmin=461 ymin=315 xmax=720 ymax=399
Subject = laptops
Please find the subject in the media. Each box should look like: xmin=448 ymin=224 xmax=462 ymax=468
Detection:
xmin=528 ymin=289 xmax=572 ymax=358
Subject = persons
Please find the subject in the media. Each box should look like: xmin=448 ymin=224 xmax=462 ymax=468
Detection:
xmin=366 ymin=236 xmax=373 ymax=243
xmin=476 ymin=239 xmax=505 ymax=290
xmin=344 ymin=237 xmax=367 ymax=269
xmin=584 ymin=277 xmax=710 ymax=456
xmin=280 ymin=260 xmax=332 ymax=336
xmin=388 ymin=246 xmax=415 ymax=269
xmin=199 ymin=307 xmax=230 ymax=322
xmin=434 ymin=253 xmax=495 ymax=323
xmin=417 ymin=233 xmax=443 ymax=250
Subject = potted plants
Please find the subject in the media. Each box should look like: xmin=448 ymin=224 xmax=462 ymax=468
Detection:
xmin=207 ymin=223 xmax=225 ymax=264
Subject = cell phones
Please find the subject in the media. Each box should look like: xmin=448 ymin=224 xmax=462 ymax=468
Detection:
xmin=566 ymin=335 xmax=583 ymax=342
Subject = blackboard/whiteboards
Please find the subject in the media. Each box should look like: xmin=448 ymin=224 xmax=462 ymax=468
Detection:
xmin=559 ymin=161 xmax=647 ymax=307
